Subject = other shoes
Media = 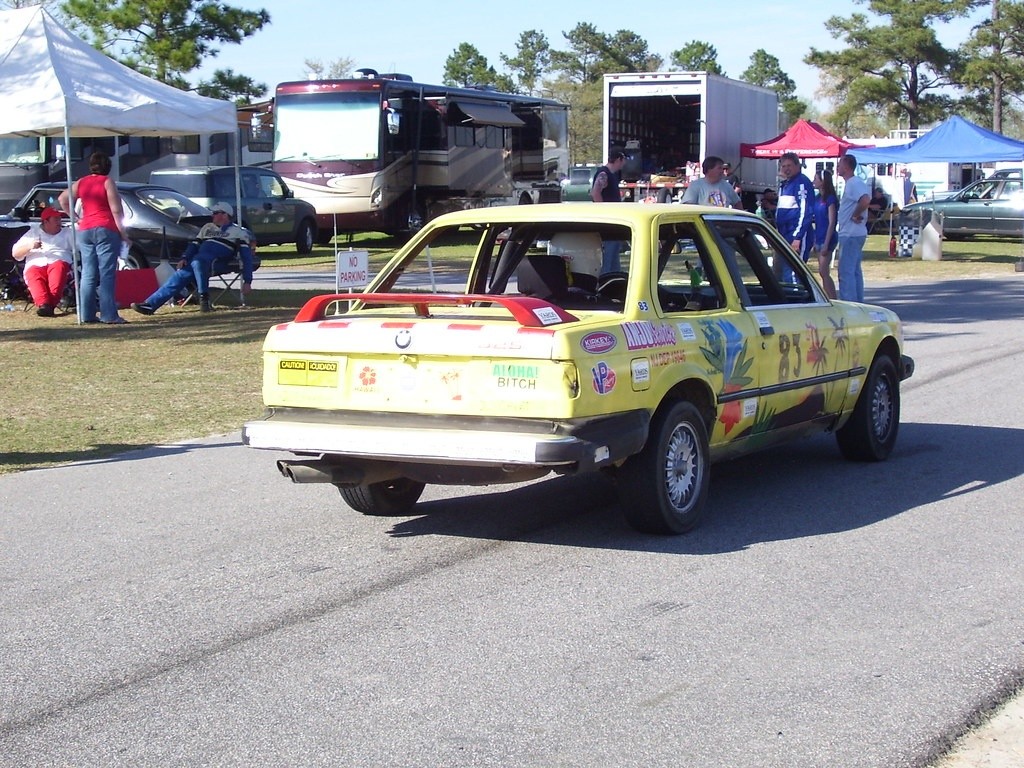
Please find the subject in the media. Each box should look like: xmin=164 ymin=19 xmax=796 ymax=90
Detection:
xmin=37 ymin=304 xmax=54 ymax=317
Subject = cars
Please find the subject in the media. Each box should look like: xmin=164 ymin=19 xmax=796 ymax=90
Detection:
xmin=240 ymin=202 xmax=914 ymax=536
xmin=562 ymin=166 xmax=599 ymax=201
xmin=0 ymin=180 xmax=261 ymax=274
xmin=896 ymin=168 xmax=1024 ymax=239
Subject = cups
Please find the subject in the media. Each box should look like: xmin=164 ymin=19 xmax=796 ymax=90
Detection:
xmin=34 ymin=233 xmax=41 ymax=243
xmin=120 ymin=240 xmax=129 ymax=260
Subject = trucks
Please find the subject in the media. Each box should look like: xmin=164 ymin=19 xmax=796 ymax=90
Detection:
xmin=602 ymin=69 xmax=786 ymax=204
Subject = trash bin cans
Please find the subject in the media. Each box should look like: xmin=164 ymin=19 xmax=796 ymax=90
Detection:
xmin=922 ymin=209 xmax=946 ymax=261
xmin=897 ymin=209 xmax=931 ymax=257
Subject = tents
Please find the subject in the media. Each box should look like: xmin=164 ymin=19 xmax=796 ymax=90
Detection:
xmin=0 ymin=4 xmax=245 ymax=325
xmin=846 ymin=115 xmax=1024 ymax=257
xmin=739 ymin=118 xmax=876 ymax=222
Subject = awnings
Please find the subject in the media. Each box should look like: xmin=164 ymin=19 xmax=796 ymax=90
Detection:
xmin=451 ymin=102 xmax=527 ymax=127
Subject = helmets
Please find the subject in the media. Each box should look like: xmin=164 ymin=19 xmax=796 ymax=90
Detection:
xmin=547 ymin=231 xmax=603 ymax=280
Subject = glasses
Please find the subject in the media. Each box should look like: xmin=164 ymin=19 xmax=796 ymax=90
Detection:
xmin=213 ymin=211 xmax=224 ymax=215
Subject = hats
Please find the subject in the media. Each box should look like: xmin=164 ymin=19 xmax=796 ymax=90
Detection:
xmin=40 ymin=207 xmax=68 ymax=221
xmin=206 ymin=202 xmax=233 ymax=218
xmin=723 ymin=162 xmax=732 ymax=169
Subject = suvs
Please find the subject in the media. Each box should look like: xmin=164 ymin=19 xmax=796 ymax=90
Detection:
xmin=148 ymin=165 xmax=319 ymax=254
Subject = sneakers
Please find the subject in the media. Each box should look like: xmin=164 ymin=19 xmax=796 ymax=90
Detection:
xmin=200 ymin=293 xmax=210 ymax=312
xmin=131 ymin=302 xmax=153 ymax=316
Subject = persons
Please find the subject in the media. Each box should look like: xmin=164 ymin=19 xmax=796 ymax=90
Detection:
xmin=837 ymin=154 xmax=871 ymax=303
xmin=681 ymin=156 xmax=743 ymax=283
xmin=761 ymin=153 xmax=839 ymax=300
xmin=58 ymin=152 xmax=133 ymax=325
xmin=591 ymin=151 xmax=630 ymax=276
xmin=130 ymin=202 xmax=253 ymax=315
xmin=866 ymin=187 xmax=888 ymax=234
xmin=12 ymin=207 xmax=80 ymax=317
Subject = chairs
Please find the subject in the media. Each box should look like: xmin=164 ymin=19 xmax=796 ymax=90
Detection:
xmin=11 ymin=259 xmax=82 ymax=313
xmin=517 ymin=254 xmax=587 ymax=311
xmin=167 ymin=237 xmax=254 ymax=307
xmin=245 ymin=179 xmax=260 ymax=197
xmin=865 ymin=194 xmax=892 ymax=234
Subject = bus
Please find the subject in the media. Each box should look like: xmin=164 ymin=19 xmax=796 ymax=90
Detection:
xmin=250 ymin=68 xmax=573 ymax=252
